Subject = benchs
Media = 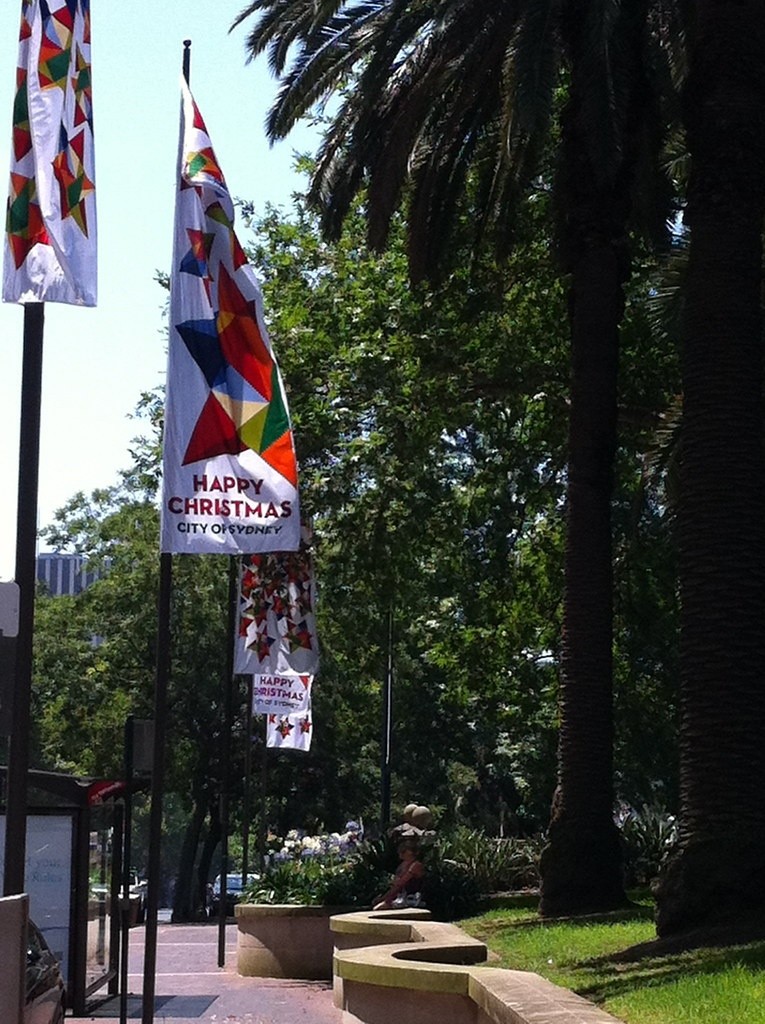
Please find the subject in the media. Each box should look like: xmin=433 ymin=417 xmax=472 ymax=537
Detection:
xmin=327 ymin=899 xmax=623 ymax=1024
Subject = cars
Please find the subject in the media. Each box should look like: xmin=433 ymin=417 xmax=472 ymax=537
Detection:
xmin=208 ymin=873 xmax=252 ymax=917
xmin=116 ymin=865 xmax=148 ymax=930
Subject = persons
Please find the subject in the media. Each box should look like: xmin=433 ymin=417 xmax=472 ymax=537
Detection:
xmin=157 ymin=66 xmax=300 ymax=556
xmin=373 ymin=838 xmax=425 ymax=910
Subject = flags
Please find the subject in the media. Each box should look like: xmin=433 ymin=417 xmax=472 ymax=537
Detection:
xmin=266 ymin=710 xmax=312 ymax=751
xmin=251 ymin=673 xmax=314 ymax=713
xmin=233 ymin=509 xmax=319 ymax=674
xmin=1 ymin=0 xmax=97 ymax=310
